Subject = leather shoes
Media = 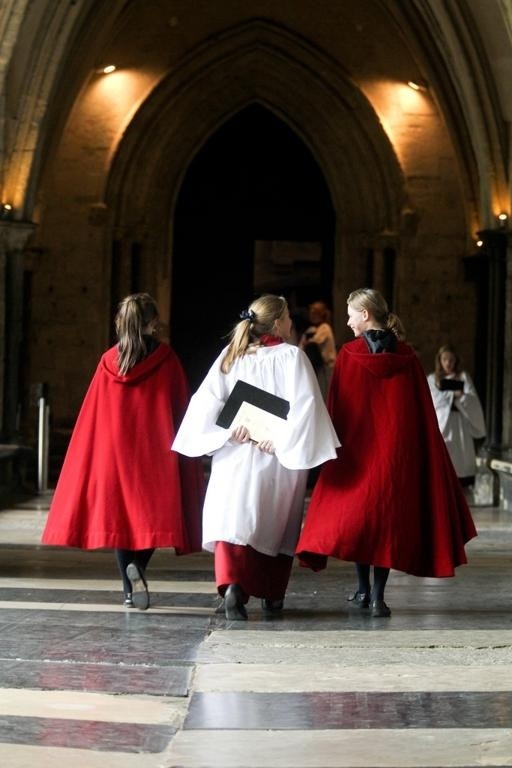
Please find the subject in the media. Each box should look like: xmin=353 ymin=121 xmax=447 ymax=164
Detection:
xmin=124 ymin=562 xmax=150 ymax=610
xmin=223 ymin=583 xmax=248 ymax=621
xmin=261 ymin=598 xmax=283 ymax=612
xmin=347 ymin=591 xmax=391 ymax=617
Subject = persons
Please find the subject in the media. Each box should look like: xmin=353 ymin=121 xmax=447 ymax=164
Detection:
xmin=298 ymin=302 xmax=337 ymax=371
xmin=303 ymin=342 xmax=331 ymax=403
xmin=295 ymin=288 xmax=477 ymax=617
xmin=170 ymin=294 xmax=341 ymax=620
xmin=426 ymin=344 xmax=486 ymax=487
xmin=41 ymin=293 xmax=205 ymax=610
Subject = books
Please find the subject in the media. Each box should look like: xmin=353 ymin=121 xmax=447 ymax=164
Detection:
xmin=215 ymin=380 xmax=290 ymax=449
xmin=440 ymin=379 xmax=464 ymax=411
